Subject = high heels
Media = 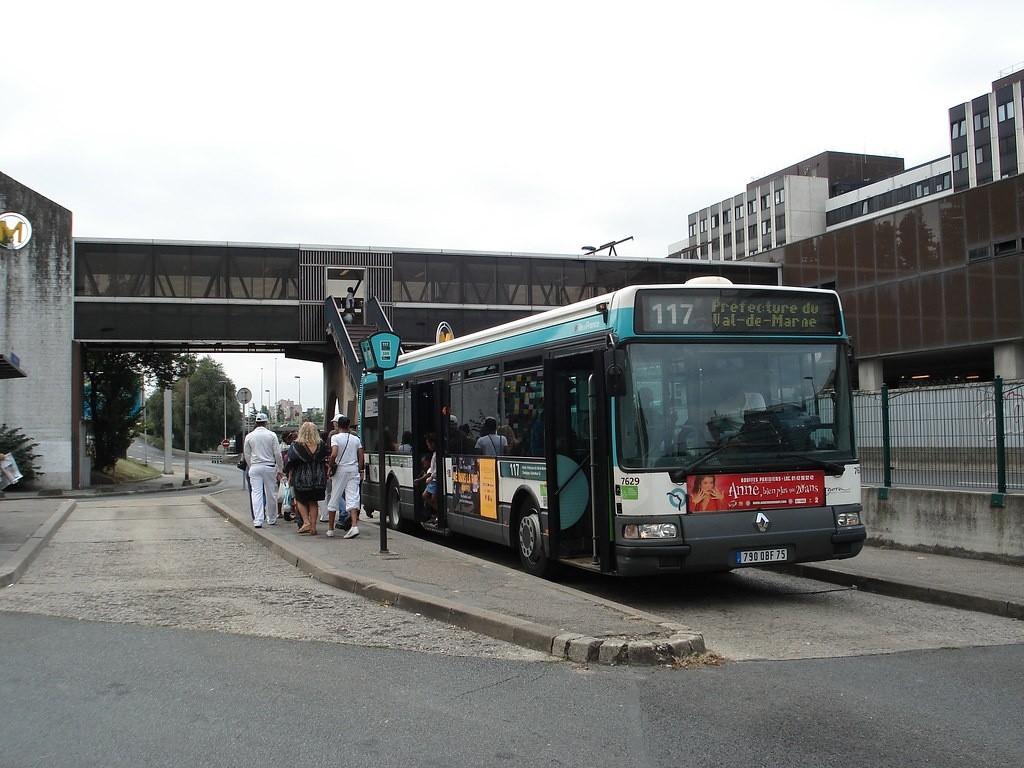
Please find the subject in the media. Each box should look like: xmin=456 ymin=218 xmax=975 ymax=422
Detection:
xmin=310 ymin=529 xmax=317 ymax=535
xmin=298 ymin=522 xmax=311 ymax=533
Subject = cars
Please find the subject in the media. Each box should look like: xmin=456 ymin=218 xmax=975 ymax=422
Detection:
xmin=230 ymin=438 xmax=235 ymax=443
xmin=818 ymin=389 xmax=834 ymax=399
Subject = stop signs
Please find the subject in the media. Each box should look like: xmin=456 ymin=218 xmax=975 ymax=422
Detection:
xmin=222 ymin=440 xmax=229 ymax=448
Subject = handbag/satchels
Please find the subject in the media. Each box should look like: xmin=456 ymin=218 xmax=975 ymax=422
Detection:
xmin=277 ymin=480 xmax=295 ymax=511
xmin=237 ymin=458 xmax=246 ymax=471
xmin=329 ymin=463 xmax=338 ymax=476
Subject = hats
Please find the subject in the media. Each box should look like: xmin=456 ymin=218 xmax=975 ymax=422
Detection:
xmin=338 ymin=417 xmax=350 ymax=427
xmin=450 ymin=415 xmax=457 ymax=423
xmin=256 ymin=414 xmax=268 ymax=422
xmin=331 ymin=413 xmax=345 ymax=422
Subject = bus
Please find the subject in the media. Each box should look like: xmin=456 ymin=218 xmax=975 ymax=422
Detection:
xmin=358 ymin=277 xmax=867 ymax=577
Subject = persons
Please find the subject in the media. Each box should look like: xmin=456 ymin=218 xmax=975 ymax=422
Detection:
xmin=715 ymin=385 xmax=766 ymax=426
xmin=345 ymin=287 xmax=355 ymax=326
xmin=373 ymin=429 xmax=412 ymax=455
xmin=448 ymin=414 xmax=545 ymax=457
xmin=416 ymin=454 xmax=432 ymax=486
xmin=414 ymin=433 xmax=438 ymax=529
xmin=244 ymin=412 xmax=364 ymax=539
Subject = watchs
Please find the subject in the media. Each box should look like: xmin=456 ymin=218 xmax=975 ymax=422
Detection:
xmin=360 ymin=470 xmax=364 ymax=472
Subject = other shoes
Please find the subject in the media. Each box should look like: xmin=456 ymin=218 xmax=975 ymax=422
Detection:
xmin=343 ymin=514 xmax=352 ymax=531
xmin=273 ymin=511 xmax=303 ymax=528
xmin=335 ymin=523 xmax=345 ymax=530
xmin=325 ymin=530 xmax=334 ymax=537
xmin=344 ymin=526 xmax=360 ymax=539
xmin=427 ymin=515 xmax=438 ymax=523
xmin=255 ymin=524 xmax=262 ymax=528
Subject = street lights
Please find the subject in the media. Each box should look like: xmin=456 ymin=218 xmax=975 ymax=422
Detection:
xmin=275 ymin=358 xmax=277 ymax=417
xmin=295 ymin=376 xmax=302 ymax=426
xmin=261 ymin=368 xmax=263 ymax=406
xmin=219 ymin=380 xmax=227 ymax=455
xmin=265 ymin=390 xmax=270 ymax=430
xmin=804 ymin=376 xmax=819 ymax=416
